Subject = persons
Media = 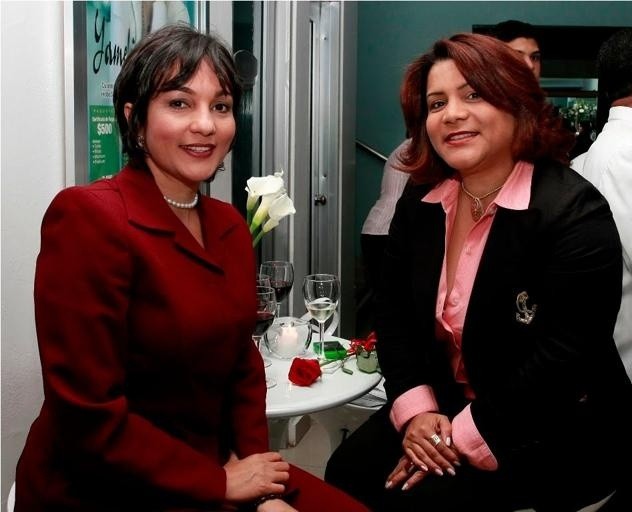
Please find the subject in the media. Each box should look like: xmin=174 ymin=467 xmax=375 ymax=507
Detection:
xmin=355 ymin=135 xmax=424 ymax=310
xmin=570 ymin=31 xmax=632 ymax=381
xmin=10 ymin=18 xmax=375 ymax=510
xmin=489 ymin=19 xmax=541 ymax=84
xmin=160 ymin=194 xmax=200 ymax=209
xmin=323 ymin=32 xmax=632 ymax=509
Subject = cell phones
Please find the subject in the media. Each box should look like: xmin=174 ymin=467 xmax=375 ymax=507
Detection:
xmin=314 ymin=341 xmax=346 ymax=359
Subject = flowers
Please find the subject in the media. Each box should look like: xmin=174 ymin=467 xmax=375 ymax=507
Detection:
xmin=288 ymin=331 xmax=383 ymax=387
xmin=243 ymin=171 xmax=298 ymax=249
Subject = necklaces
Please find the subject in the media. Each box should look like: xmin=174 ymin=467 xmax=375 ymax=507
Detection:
xmin=459 ymin=178 xmax=503 ymax=222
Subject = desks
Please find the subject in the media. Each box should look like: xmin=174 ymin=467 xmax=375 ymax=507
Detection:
xmin=254 ymin=333 xmax=383 ymax=453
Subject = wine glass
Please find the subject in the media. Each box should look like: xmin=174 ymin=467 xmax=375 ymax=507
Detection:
xmin=250 ymin=274 xmax=278 ymax=389
xmin=259 ymin=261 xmax=295 ymax=317
xmin=301 ymin=273 xmax=342 ymax=369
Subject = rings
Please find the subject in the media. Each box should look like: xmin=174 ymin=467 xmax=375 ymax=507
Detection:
xmin=427 ymin=433 xmax=441 ymax=447
